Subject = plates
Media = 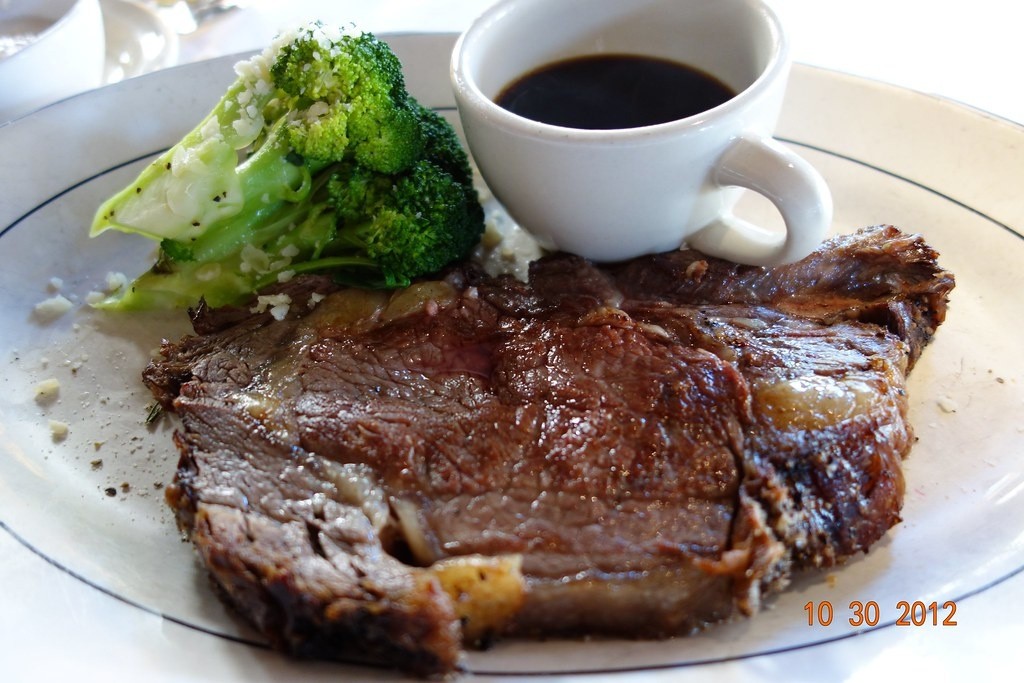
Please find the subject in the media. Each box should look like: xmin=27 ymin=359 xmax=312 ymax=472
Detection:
xmin=0 ymin=32 xmax=1024 ymax=680
xmin=110 ymin=0 xmax=176 ymax=82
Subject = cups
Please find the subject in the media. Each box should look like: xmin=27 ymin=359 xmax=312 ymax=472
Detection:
xmin=449 ymin=0 xmax=831 ymax=267
xmin=0 ymin=0 xmax=104 ymax=131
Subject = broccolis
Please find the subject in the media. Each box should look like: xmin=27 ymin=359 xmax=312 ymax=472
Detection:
xmin=87 ymin=18 xmax=485 ymax=321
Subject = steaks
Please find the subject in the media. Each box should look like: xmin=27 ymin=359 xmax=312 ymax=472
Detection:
xmin=140 ymin=223 xmax=955 ymax=683
